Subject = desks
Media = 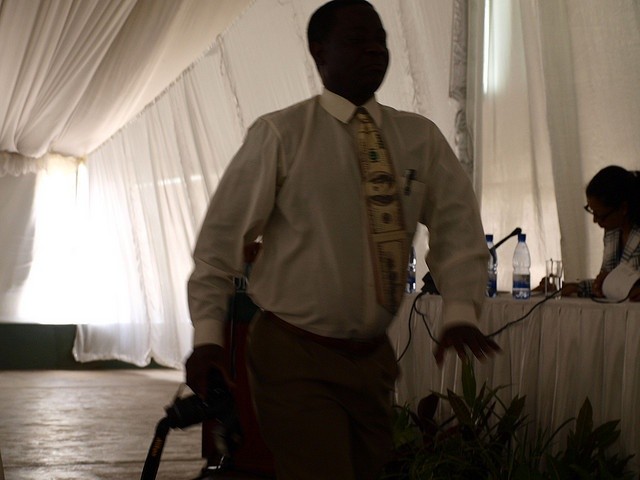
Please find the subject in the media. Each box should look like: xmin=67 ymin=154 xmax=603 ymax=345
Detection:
xmin=387 ymin=293 xmax=640 ymax=459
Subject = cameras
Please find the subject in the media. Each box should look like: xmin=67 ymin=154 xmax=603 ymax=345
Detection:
xmin=165 ymin=374 xmax=229 ymax=431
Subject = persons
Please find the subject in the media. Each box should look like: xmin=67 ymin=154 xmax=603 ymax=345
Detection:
xmin=184 ymin=0 xmax=506 ymax=480
xmin=539 ymin=165 xmax=640 ymax=303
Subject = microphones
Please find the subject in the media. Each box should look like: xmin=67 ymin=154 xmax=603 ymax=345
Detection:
xmin=490 ymin=228 xmax=521 ymax=252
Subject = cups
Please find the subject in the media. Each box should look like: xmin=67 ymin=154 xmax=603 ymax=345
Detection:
xmin=545 ymin=260 xmax=563 ymax=301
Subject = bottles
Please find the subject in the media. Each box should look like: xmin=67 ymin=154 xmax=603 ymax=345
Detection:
xmin=405 ymin=246 xmax=417 ymax=294
xmin=486 ymin=234 xmax=497 ymax=298
xmin=513 ymin=234 xmax=531 ymax=303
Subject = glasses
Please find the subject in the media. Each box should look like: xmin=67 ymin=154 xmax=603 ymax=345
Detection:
xmin=584 ymin=203 xmax=598 ymax=216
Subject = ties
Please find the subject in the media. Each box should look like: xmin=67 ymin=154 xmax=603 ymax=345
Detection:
xmin=349 ymin=110 xmax=413 ymax=313
